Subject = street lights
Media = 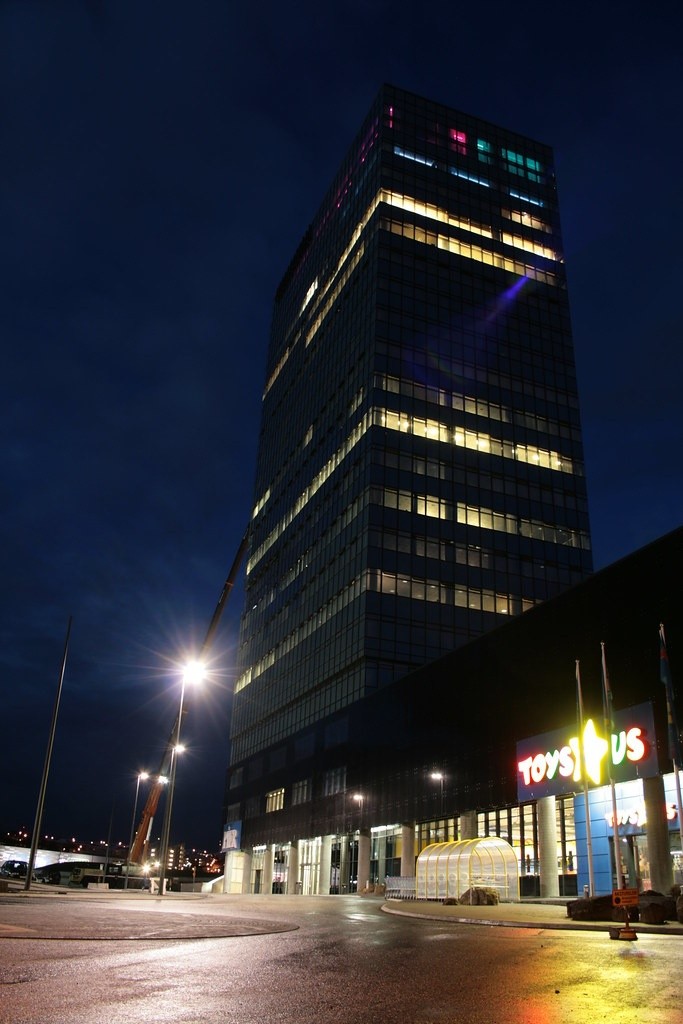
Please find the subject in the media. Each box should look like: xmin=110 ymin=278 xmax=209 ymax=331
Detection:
xmin=124 ymin=772 xmax=149 ymax=889
xmin=157 ymin=662 xmax=206 ymax=896
xmin=157 ymin=744 xmax=187 ymax=878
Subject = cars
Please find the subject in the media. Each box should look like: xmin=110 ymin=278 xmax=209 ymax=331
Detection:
xmin=0 ymin=860 xmax=35 ymax=882
xmin=33 ymin=867 xmax=61 ymax=885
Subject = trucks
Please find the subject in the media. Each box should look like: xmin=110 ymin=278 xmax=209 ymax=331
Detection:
xmin=68 ymin=868 xmax=105 ymax=885
